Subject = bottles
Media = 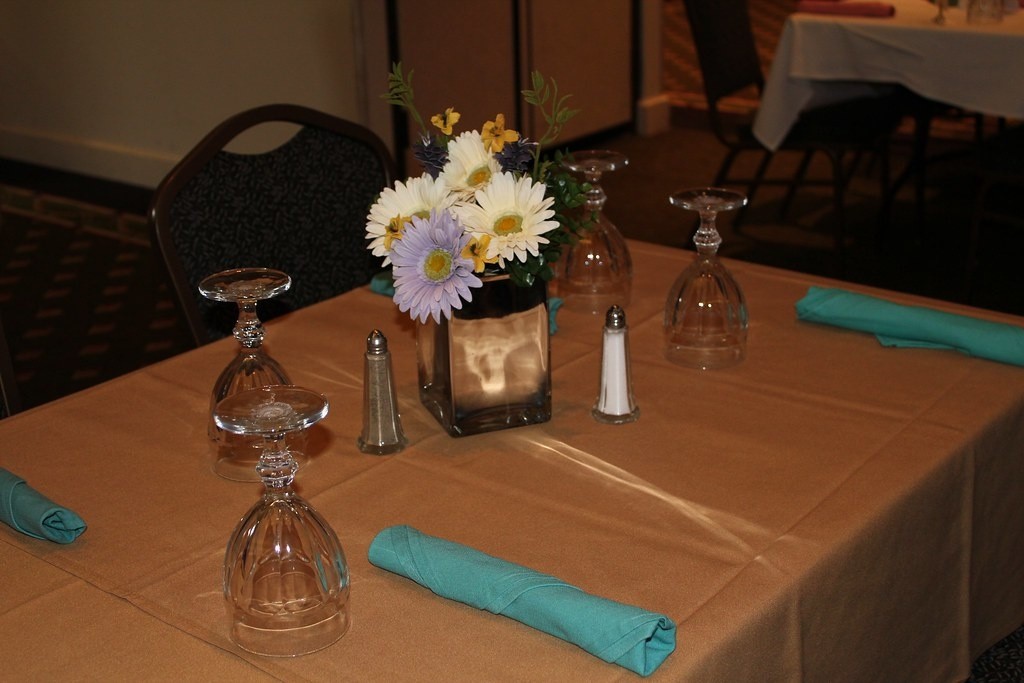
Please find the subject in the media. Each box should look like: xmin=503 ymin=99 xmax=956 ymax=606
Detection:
xmin=357 ymin=328 xmax=409 ymax=454
xmin=592 ymin=303 xmax=641 ymax=425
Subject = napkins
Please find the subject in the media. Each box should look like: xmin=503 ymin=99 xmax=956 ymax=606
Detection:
xmin=365 ymin=526 xmax=678 ymax=677
xmin=0 ymin=468 xmax=88 ymax=546
xmin=793 ymin=285 xmax=1024 ymax=367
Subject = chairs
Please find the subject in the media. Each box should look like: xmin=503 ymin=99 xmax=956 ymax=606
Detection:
xmin=150 ymin=102 xmax=395 ymax=348
xmin=683 ymin=0 xmax=897 ymax=265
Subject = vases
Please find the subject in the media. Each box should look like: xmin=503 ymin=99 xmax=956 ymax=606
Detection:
xmin=417 ymin=269 xmax=552 ymax=437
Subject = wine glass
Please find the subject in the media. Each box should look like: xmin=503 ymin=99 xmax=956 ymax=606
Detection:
xmin=196 ymin=267 xmax=310 ymax=482
xmin=663 ymin=185 xmax=749 ymax=373
xmin=558 ymin=150 xmax=633 ymax=294
xmin=212 ymin=384 xmax=352 ymax=656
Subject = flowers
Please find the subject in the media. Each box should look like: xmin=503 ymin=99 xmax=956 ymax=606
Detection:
xmin=362 ymin=63 xmax=601 ymax=325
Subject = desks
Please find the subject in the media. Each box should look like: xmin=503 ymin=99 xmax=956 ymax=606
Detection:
xmin=750 ymin=0 xmax=1024 ymax=273
xmin=0 ymin=240 xmax=1009 ymax=683
